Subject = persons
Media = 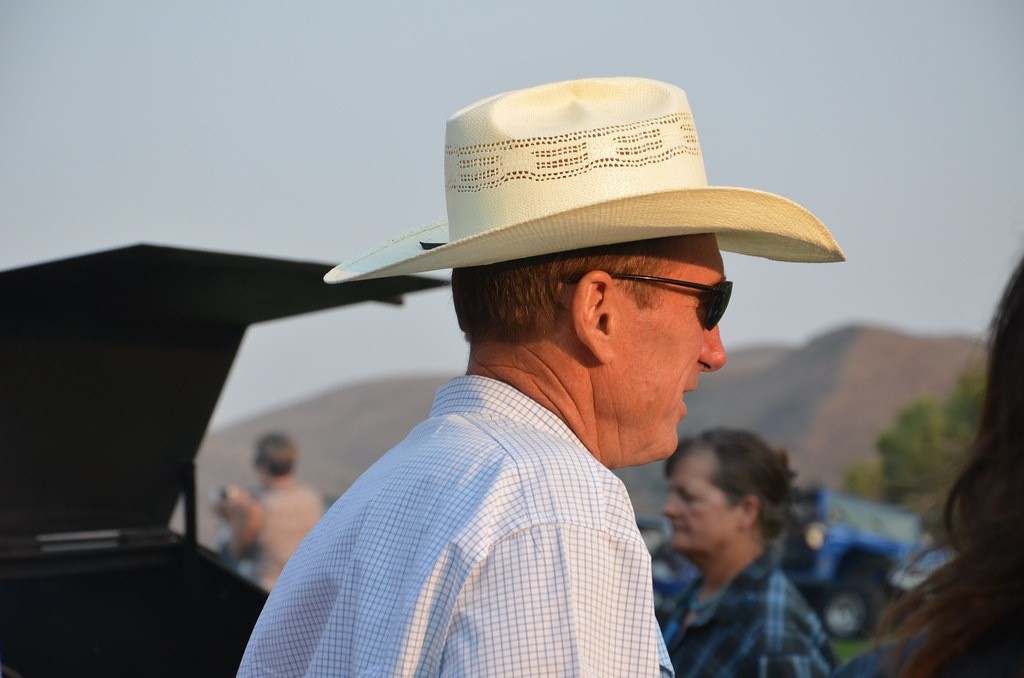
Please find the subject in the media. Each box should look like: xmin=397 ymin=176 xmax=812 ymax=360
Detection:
xmin=832 ymin=258 xmax=1024 ymax=678
xmin=217 ymin=434 xmax=324 ymax=593
xmin=237 ymin=76 xmax=847 ymax=678
xmin=652 ymin=428 xmax=839 ymax=678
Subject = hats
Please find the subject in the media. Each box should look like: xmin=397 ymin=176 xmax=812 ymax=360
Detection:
xmin=323 ymin=76 xmax=846 ymax=283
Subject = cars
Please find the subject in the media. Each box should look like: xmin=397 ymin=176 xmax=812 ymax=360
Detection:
xmin=0 ymin=239 xmax=451 ymax=677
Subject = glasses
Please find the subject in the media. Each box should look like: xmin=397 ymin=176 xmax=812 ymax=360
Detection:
xmin=568 ymin=273 xmax=732 ymax=332
